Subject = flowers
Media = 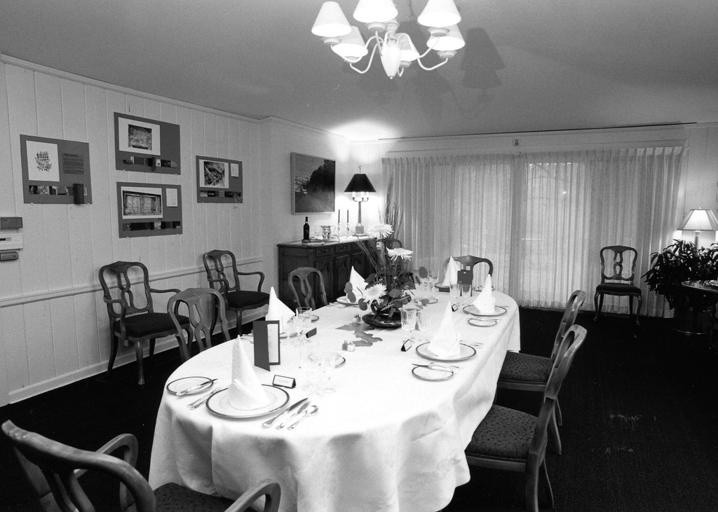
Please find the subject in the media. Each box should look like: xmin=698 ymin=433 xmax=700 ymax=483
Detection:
xmin=367 ymin=221 xmax=412 ymax=314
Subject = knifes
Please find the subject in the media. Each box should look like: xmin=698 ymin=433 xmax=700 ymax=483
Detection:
xmin=260 ymin=395 xmax=318 ymax=429
xmin=187 ymin=387 xmax=218 ymax=409
xmin=175 ymin=378 xmax=218 ymax=395
xmin=410 ymin=363 xmax=450 ymax=373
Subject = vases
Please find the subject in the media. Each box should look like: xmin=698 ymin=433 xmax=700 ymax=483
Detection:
xmin=362 ymin=307 xmax=408 ymax=329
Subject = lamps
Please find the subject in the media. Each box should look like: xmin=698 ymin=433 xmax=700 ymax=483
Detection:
xmin=341 ymin=172 xmax=379 ymax=239
xmin=675 ymin=207 xmax=718 ymax=257
xmin=311 ymin=1 xmax=469 ymax=82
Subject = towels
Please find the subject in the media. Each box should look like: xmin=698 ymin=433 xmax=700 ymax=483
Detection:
xmin=477 ymin=273 xmax=498 ymax=315
xmin=260 ymin=283 xmax=300 ymax=334
xmin=426 ymin=300 xmax=462 ymax=362
xmin=344 ymin=262 xmax=373 ymax=306
xmin=444 ymin=255 xmax=464 ymax=289
xmin=226 ymin=331 xmax=277 ymax=415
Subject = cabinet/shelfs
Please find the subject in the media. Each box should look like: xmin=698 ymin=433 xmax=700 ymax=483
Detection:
xmin=277 ymin=236 xmax=379 ymax=314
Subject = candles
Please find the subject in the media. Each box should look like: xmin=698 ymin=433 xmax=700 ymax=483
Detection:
xmin=336 ymin=209 xmax=349 ymax=224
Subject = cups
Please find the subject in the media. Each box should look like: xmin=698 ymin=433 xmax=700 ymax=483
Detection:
xmin=295 ymin=307 xmax=312 ymax=333
xmin=449 ymin=283 xmax=462 ymax=304
xmin=315 ymin=353 xmax=336 ymax=391
xmin=400 ymin=307 xmax=417 ymax=332
xmin=462 ymin=284 xmax=471 ymax=300
xmin=322 ymin=225 xmax=331 ymax=242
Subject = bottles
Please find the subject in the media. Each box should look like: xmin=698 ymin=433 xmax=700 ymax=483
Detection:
xmin=303 ymin=216 xmax=310 ymax=240
xmin=414 ymin=308 xmax=422 ymax=330
xmin=423 ymin=281 xmax=433 ymax=301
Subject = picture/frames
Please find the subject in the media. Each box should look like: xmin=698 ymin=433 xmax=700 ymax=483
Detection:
xmin=288 ymin=152 xmax=337 ymax=215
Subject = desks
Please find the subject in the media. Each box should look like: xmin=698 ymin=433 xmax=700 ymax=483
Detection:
xmin=679 ymin=277 xmax=717 ymax=335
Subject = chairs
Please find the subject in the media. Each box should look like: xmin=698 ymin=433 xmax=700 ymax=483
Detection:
xmin=495 ymin=289 xmax=588 ymax=459
xmin=591 ymin=244 xmax=643 ymax=328
xmin=440 ymin=253 xmax=497 ymax=300
xmin=460 ymin=320 xmax=595 ymax=510
xmin=202 ymin=248 xmax=274 ymax=342
xmin=163 ymin=285 xmax=235 ymax=366
xmin=283 ymin=264 xmax=329 ymax=316
xmin=1 ymin=414 xmax=286 ymax=511
xmin=96 ymin=260 xmax=194 ymax=390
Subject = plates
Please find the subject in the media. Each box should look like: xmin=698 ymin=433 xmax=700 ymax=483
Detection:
xmin=336 ymin=296 xmax=370 ymax=305
xmin=307 ymin=351 xmax=347 ymax=368
xmin=462 ymin=305 xmax=507 ymax=315
xmin=412 ymin=366 xmax=453 ymax=381
xmin=415 ymin=341 xmax=477 ymax=362
xmin=204 ymin=382 xmax=290 ymax=420
xmin=468 ymin=316 xmax=496 ymax=327
xmin=311 ymin=315 xmax=319 ymax=321
xmin=166 ymin=376 xmax=213 ymax=396
